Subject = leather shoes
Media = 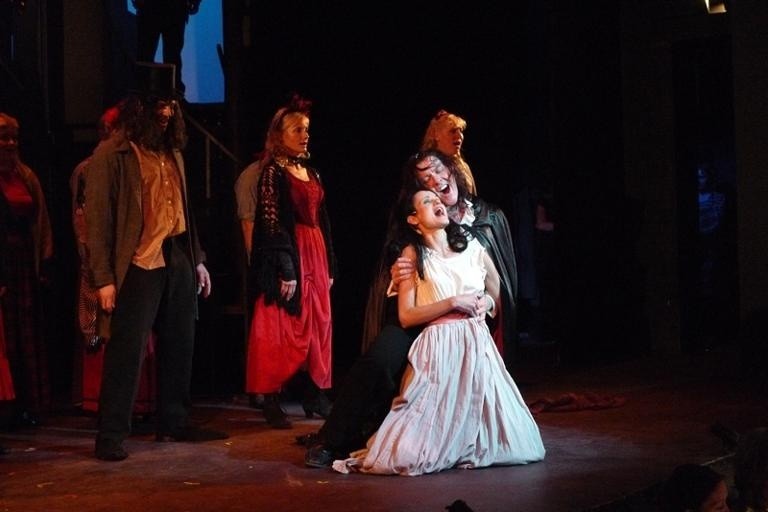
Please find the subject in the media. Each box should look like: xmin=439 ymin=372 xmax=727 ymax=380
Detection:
xmin=304 ymin=440 xmax=334 ymax=470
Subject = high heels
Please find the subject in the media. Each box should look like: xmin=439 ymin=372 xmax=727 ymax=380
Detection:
xmin=93 ymin=433 xmax=131 ymax=462
xmin=301 ymin=388 xmax=336 ymax=422
xmin=153 ymin=421 xmax=233 ymax=444
xmin=260 ymin=394 xmax=294 ymax=430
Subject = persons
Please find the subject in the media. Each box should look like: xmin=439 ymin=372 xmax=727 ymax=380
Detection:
xmin=83 ymin=77 xmax=231 ymax=461
xmin=331 ymin=187 xmax=546 ymax=477
xmin=131 ymin=0 xmax=203 ymax=103
xmin=654 ymin=462 xmax=732 ymax=512
xmin=1 ymin=111 xmax=54 ymax=431
xmin=422 ymin=109 xmax=477 ymax=196
xmin=234 ymin=105 xmax=340 ymax=427
xmin=293 ymin=149 xmax=519 ymax=467
xmin=68 ymin=99 xmax=161 ymax=413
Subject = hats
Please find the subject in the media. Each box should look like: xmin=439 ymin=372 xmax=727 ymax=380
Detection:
xmin=123 ymin=58 xmax=186 ymax=102
